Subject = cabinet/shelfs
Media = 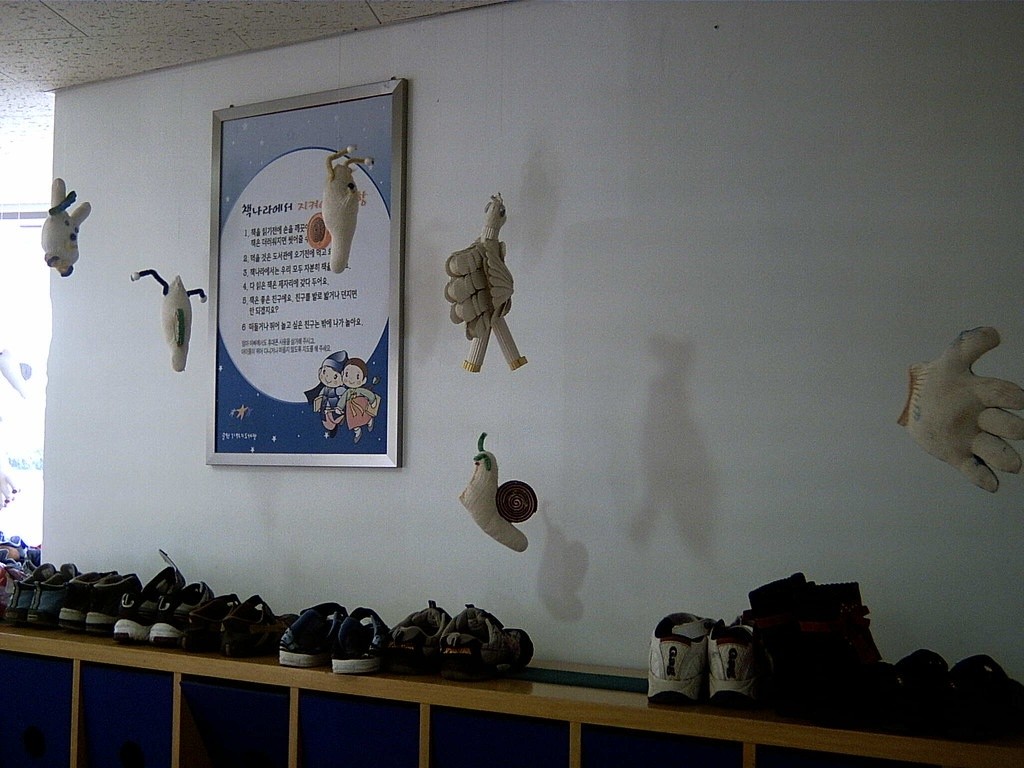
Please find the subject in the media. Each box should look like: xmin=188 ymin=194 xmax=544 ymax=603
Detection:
xmin=0 ymin=625 xmax=1024 ymax=768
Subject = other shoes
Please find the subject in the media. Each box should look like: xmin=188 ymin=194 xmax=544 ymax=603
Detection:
xmin=279 ymin=602 xmax=350 ymax=667
xmin=180 ymin=592 xmax=244 ymax=651
xmin=330 ymin=608 xmax=392 ymax=672
xmin=882 ymin=649 xmax=952 ymax=738
xmin=85 ymin=573 xmax=143 ymax=631
xmin=24 ymin=564 xmax=79 ymax=622
xmin=0 ymin=529 xmax=57 ymax=622
xmin=220 ymin=593 xmax=300 ymax=658
xmin=946 ymin=653 xmax=1024 ymax=745
xmin=58 ymin=570 xmax=119 ymax=630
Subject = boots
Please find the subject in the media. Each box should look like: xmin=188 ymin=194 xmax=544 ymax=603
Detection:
xmin=747 ymin=573 xmax=895 ymax=725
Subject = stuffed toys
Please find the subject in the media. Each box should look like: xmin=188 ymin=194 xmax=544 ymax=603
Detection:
xmin=445 ymin=193 xmax=527 ymax=373
xmin=460 ymin=434 xmax=538 ymax=553
xmin=323 ymin=145 xmax=374 ymax=274
xmin=41 ymin=178 xmax=91 ymax=278
xmin=130 ymin=270 xmax=208 ymax=372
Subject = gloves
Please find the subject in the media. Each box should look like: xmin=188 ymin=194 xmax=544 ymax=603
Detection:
xmin=897 ymin=325 xmax=1024 ymax=492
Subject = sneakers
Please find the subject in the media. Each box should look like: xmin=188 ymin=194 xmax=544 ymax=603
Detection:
xmin=439 ymin=605 xmax=535 ymax=679
xmin=385 ymin=600 xmax=452 ymax=675
xmin=114 ymin=550 xmax=187 ymax=644
xmin=647 ymin=612 xmax=717 ymax=706
xmin=149 ymin=581 xmax=214 ymax=649
xmin=707 ymin=616 xmax=758 ymax=711
